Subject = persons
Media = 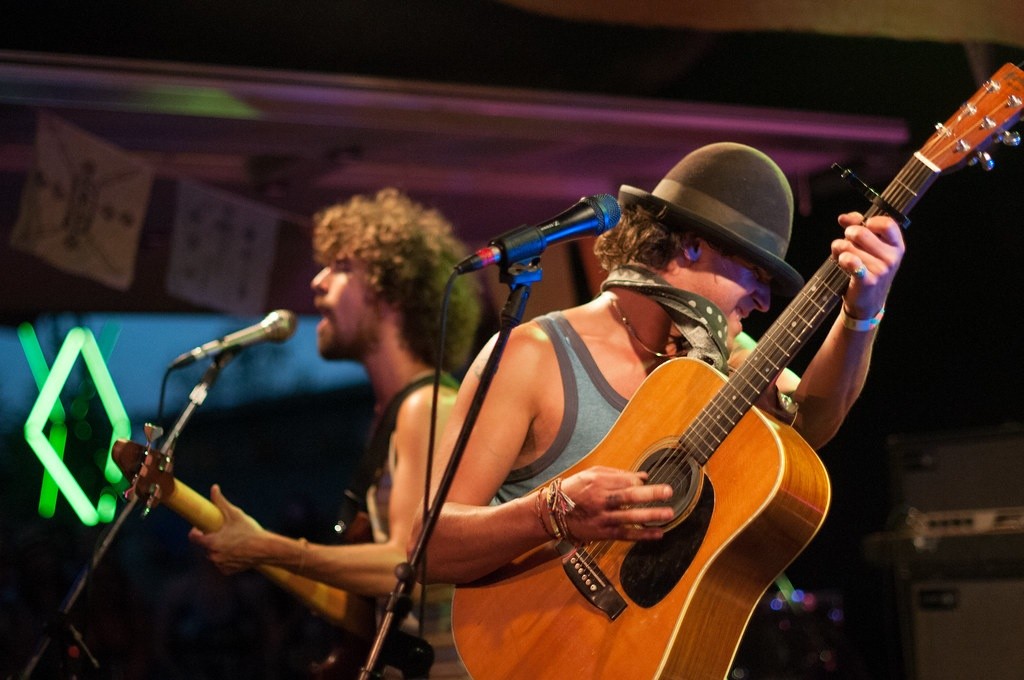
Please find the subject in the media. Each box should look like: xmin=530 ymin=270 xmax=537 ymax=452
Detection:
xmin=190 ymin=188 xmax=483 ymax=680
xmin=406 ymin=142 xmax=905 ymax=584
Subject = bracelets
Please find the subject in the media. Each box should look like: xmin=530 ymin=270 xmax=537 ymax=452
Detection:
xmin=841 ymin=303 xmax=884 ymax=331
xmin=535 ymin=478 xmax=575 ymax=540
xmin=300 ymin=538 xmax=308 ymax=569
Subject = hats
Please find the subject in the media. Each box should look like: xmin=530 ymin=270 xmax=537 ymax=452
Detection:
xmin=617 ymin=141 xmax=799 ymax=281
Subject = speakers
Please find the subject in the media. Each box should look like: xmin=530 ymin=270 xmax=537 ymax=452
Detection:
xmin=886 ymin=430 xmax=1024 ymax=680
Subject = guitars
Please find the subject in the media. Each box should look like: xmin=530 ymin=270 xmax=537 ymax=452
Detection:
xmin=452 ymin=62 xmax=1024 ymax=680
xmin=112 ymin=420 xmax=436 ymax=679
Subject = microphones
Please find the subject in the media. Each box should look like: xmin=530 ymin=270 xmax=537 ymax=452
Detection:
xmin=176 ymin=312 xmax=297 ymax=365
xmin=455 ymin=197 xmax=621 ymax=276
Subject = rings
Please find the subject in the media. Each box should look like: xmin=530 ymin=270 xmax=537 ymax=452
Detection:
xmin=851 ymin=265 xmax=867 ymax=279
